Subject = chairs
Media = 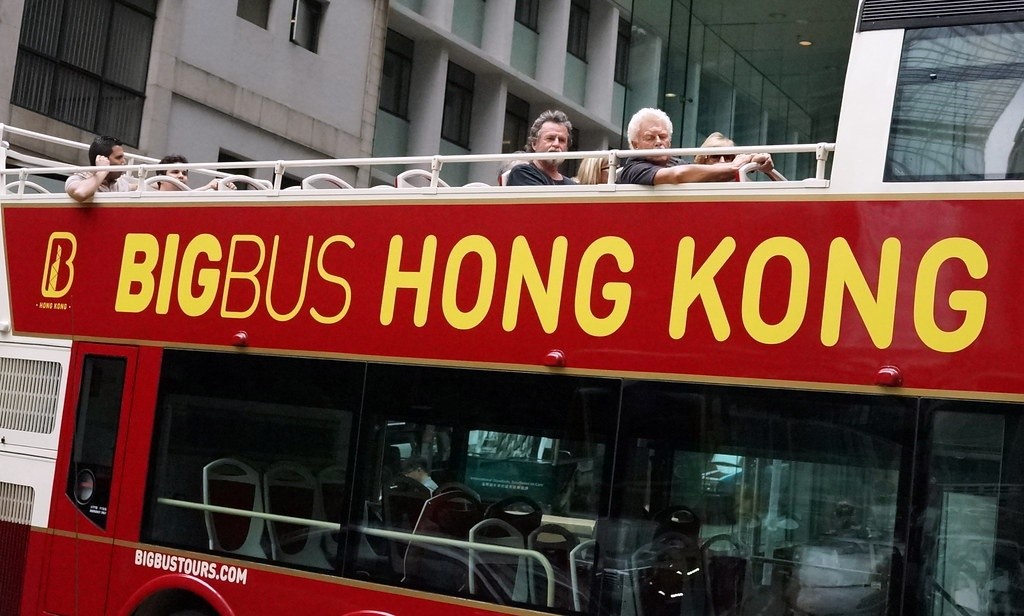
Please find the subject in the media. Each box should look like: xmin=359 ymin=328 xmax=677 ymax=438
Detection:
xmin=318 ymin=465 xmax=346 ymax=558
xmin=4 ymin=163 xmax=836 ymax=197
xmin=483 ymin=497 xmax=543 ymax=550
xmin=263 ymin=462 xmax=335 ymax=570
xmin=382 ymin=475 xmax=431 ymax=574
xmin=431 ymin=483 xmax=481 ymax=520
xmin=631 ymin=543 xmax=694 ymax=616
xmin=401 ymin=491 xmax=484 ymax=593
xmin=700 ymin=535 xmax=749 ymax=615
xmin=468 ymin=518 xmax=527 ymax=603
xmin=653 ymin=506 xmax=701 ymax=544
xmin=527 ymin=525 xmax=578 ymax=606
xmin=202 ymin=459 xmax=268 ymax=560
xmin=570 ymin=540 xmax=596 ymax=612
xmin=738 ymin=510 xmax=802 ymax=558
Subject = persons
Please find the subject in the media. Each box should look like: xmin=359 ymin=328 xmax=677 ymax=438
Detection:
xmin=402 ymin=455 xmax=438 ymax=497
xmin=499 ymin=109 xmax=774 ymax=186
xmin=64 ymin=136 xmax=237 ymax=200
xmin=820 ymin=501 xmax=869 ymax=545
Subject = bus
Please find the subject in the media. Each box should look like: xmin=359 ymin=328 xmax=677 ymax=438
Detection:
xmin=1 ymin=0 xmax=1023 ymax=614
